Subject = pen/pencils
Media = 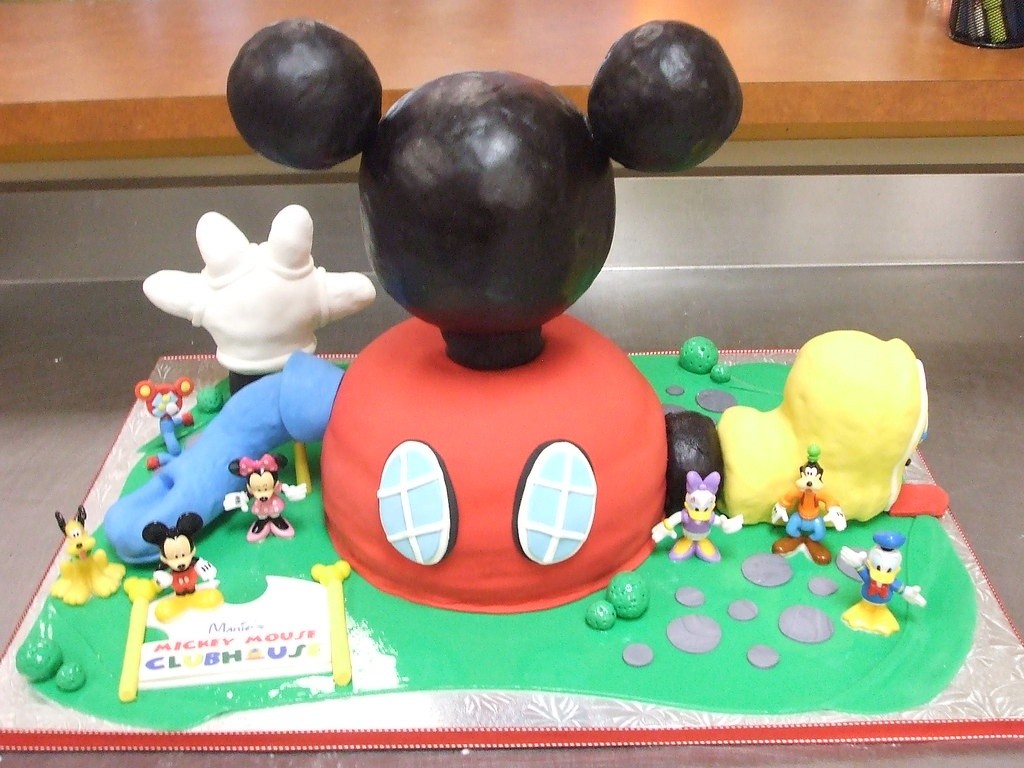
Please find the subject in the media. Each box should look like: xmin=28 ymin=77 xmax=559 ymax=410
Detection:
xmin=948 ymin=0 xmax=1024 ymax=47
